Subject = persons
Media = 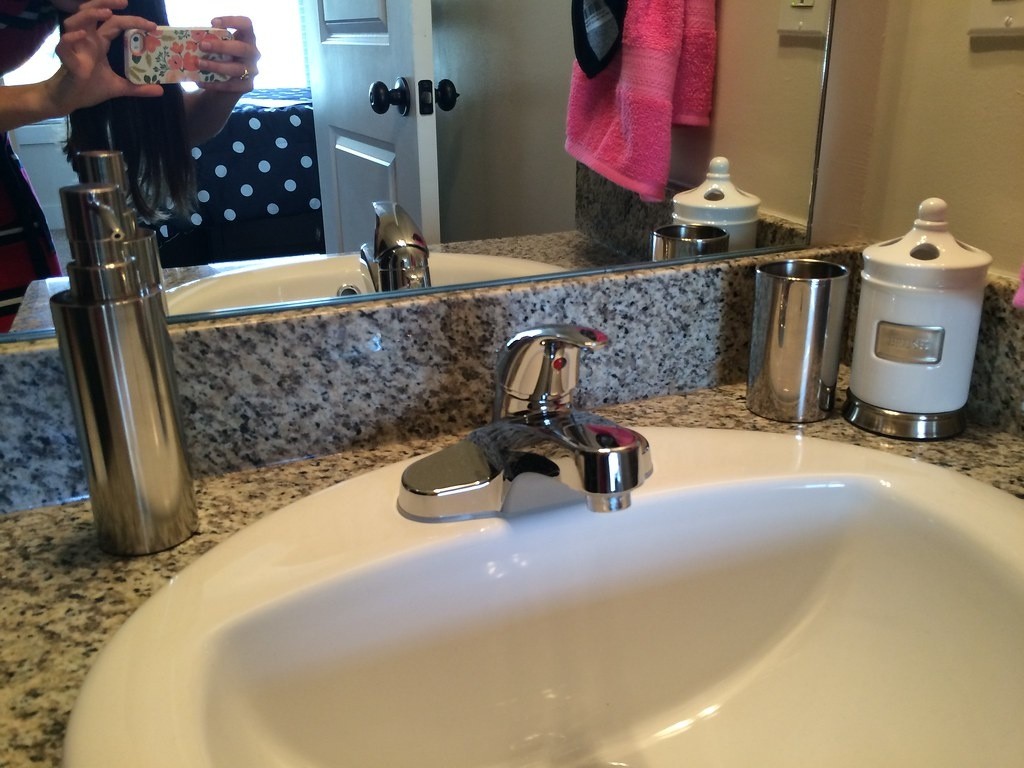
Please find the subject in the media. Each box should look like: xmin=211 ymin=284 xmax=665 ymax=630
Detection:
xmin=0 ymin=0 xmax=262 ymax=334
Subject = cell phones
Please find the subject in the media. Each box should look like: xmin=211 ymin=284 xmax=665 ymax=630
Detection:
xmin=125 ymin=25 xmax=235 ymax=86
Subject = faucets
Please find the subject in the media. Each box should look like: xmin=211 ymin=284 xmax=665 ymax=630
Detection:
xmin=358 ymin=200 xmax=432 ymax=293
xmin=396 ymin=323 xmax=654 ymax=524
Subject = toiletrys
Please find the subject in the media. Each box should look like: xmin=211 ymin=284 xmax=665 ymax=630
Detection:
xmin=49 ymin=184 xmax=202 ymax=558
xmin=75 ymin=150 xmax=171 ymax=315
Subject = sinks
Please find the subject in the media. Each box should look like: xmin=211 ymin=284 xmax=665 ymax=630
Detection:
xmin=59 ymin=423 xmax=1024 ymax=768
xmin=161 ymin=250 xmax=572 ymax=315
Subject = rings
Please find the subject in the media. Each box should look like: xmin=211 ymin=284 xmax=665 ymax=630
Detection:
xmin=239 ymin=66 xmax=249 ymax=81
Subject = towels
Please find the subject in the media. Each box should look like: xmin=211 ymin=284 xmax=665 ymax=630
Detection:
xmin=564 ymin=0 xmax=718 ymax=203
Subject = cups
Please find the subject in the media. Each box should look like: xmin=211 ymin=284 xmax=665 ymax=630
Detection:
xmin=652 ymin=224 xmax=730 ymax=263
xmin=744 ymin=259 xmax=846 ymax=423
